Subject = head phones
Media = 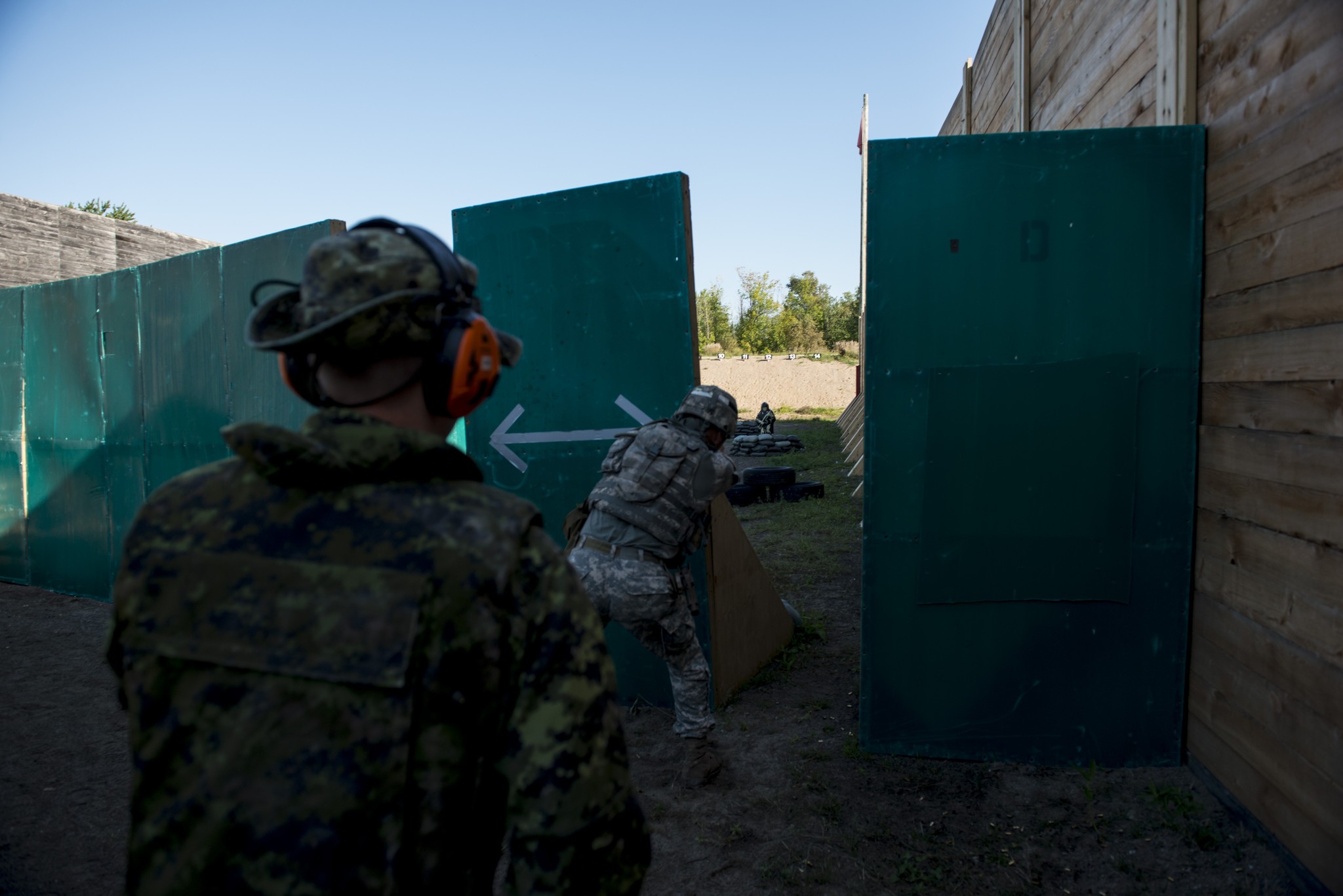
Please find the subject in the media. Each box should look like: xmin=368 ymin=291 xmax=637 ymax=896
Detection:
xmin=252 ymin=215 xmax=505 ymax=417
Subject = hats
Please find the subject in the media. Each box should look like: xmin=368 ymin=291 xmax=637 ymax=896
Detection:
xmin=243 ymin=229 xmax=526 ymax=372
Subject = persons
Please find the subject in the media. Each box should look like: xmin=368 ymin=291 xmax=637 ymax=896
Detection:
xmin=755 ymin=402 xmax=776 ymax=434
xmin=106 ymin=215 xmax=653 ymax=896
xmin=565 ymin=385 xmax=737 ymax=783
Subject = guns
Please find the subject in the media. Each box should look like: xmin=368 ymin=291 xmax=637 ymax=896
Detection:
xmin=757 ymin=410 xmax=775 ymax=430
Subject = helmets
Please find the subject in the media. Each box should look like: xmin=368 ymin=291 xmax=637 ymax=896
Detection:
xmin=674 ymin=385 xmax=740 ymax=443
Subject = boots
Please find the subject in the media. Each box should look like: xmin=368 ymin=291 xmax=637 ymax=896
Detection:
xmin=680 ymin=735 xmax=723 ymax=788
